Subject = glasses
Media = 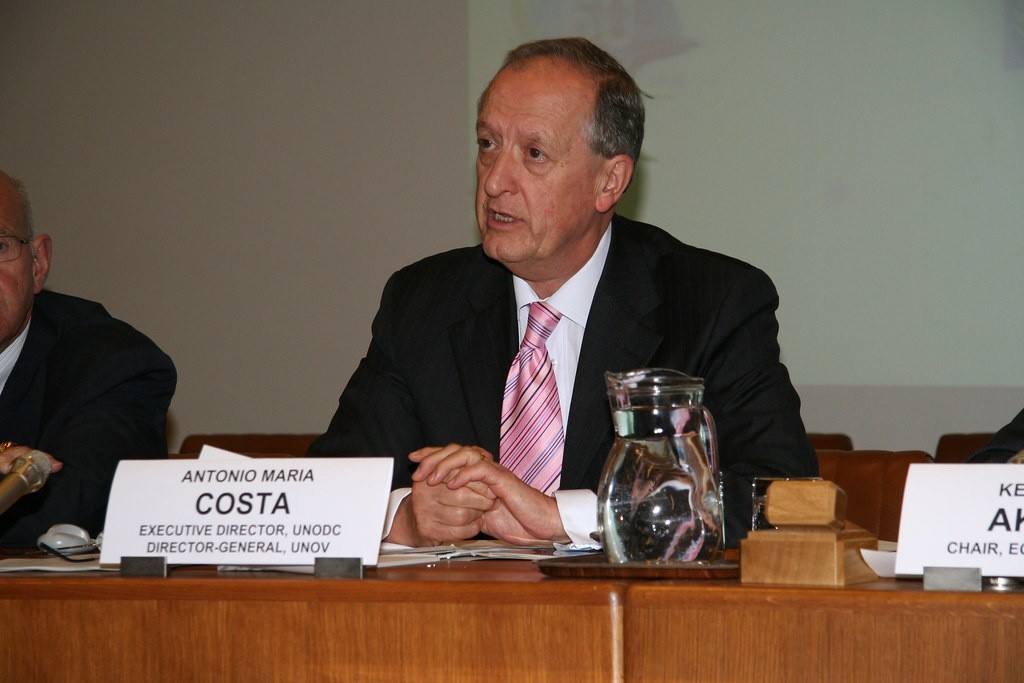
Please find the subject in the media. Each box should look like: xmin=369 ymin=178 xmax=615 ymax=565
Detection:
xmin=0 ymin=236 xmax=32 ymax=262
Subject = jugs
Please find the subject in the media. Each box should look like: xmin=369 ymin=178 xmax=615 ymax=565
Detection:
xmin=596 ymin=364 xmax=723 ymax=564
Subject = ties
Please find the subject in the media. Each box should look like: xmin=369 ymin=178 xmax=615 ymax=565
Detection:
xmin=499 ymin=301 xmax=565 ymax=496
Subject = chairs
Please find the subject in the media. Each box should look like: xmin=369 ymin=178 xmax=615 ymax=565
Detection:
xmin=179 ymin=433 xmax=319 ymax=458
xmin=807 ymin=433 xmax=993 ymax=542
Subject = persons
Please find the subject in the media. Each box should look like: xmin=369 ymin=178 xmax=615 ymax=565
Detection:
xmin=304 ymin=37 xmax=817 ymax=550
xmin=969 ymin=404 xmax=1024 ymax=464
xmin=0 ymin=169 xmax=179 ymax=556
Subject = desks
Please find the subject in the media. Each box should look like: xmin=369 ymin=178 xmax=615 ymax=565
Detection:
xmin=0 ymin=545 xmax=1024 ymax=683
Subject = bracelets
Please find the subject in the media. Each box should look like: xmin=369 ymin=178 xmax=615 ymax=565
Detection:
xmin=0 ymin=441 xmax=16 ymax=455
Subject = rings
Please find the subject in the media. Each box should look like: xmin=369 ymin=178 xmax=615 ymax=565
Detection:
xmin=481 ymin=453 xmax=486 ymax=460
